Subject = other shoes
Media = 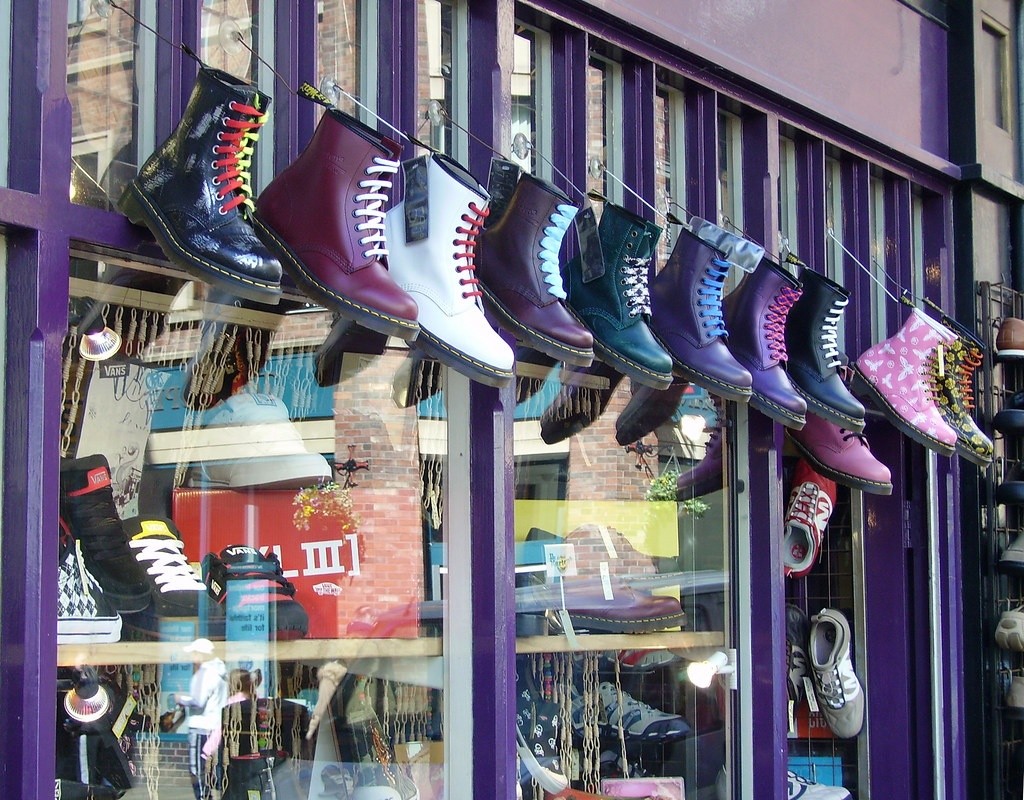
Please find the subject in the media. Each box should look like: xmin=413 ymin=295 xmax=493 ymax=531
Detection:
xmin=996 ymin=316 xmax=1024 ymax=712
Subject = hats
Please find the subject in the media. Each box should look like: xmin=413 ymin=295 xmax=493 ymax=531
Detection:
xmin=183 ymin=637 xmax=215 ymax=654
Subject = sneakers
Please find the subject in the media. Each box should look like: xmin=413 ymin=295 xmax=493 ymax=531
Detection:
xmin=121 ymin=514 xmax=208 ymax=616
xmin=57 ymin=518 xmax=122 ymax=644
xmin=514 ymin=657 xmax=567 ymax=795
xmin=807 ymin=606 xmax=865 ymax=739
xmin=546 ymin=683 xmax=611 ymax=745
xmin=60 ymin=453 xmax=152 ymax=614
xmin=548 ymin=573 xmax=688 ymax=633
xmin=200 ymin=544 xmax=308 ymax=639
xmin=784 ymin=603 xmax=810 ymax=712
xmin=713 ymin=762 xmax=854 ymax=800
xmin=584 ymin=681 xmax=691 ymax=741
xmin=781 ymin=457 xmax=838 ymax=579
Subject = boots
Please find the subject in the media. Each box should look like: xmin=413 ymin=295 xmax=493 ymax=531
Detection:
xmin=115 ymin=64 xmax=998 ymax=497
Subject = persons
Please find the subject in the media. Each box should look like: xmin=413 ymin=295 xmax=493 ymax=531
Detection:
xmin=175 ymin=639 xmax=272 ymax=800
